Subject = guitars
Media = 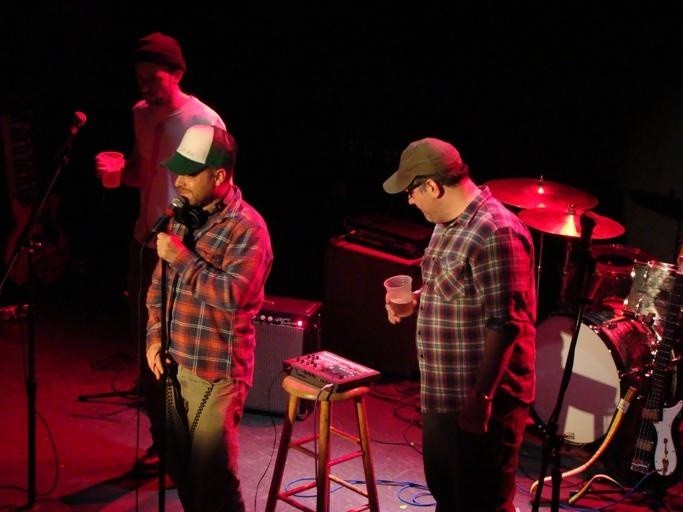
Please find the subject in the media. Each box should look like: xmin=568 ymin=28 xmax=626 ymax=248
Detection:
xmin=604 ymin=247 xmax=683 ymax=489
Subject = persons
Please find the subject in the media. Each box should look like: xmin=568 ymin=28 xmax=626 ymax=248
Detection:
xmin=93 ymin=29 xmax=227 ymax=403
xmin=382 ymin=137 xmax=537 ymax=512
xmin=144 ymin=122 xmax=273 ymax=511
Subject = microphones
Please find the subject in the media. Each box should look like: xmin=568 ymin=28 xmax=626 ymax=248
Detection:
xmin=146 ymin=194 xmax=186 ymax=240
xmin=62 ymin=111 xmax=88 ymax=157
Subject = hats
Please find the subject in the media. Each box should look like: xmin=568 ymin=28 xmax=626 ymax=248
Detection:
xmin=160 ymin=126 xmax=236 ymax=175
xmin=131 ymin=32 xmax=187 ymax=71
xmin=382 ymin=137 xmax=465 ymax=195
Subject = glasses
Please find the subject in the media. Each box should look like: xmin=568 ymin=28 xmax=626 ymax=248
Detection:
xmin=404 ymin=181 xmax=427 ymax=195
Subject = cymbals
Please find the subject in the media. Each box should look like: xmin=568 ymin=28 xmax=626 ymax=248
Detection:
xmin=478 ymin=178 xmax=598 ymax=212
xmin=517 ymin=210 xmax=626 ymax=239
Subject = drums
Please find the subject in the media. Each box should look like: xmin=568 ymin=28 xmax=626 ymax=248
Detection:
xmin=626 ymin=257 xmax=683 ymax=328
xmin=592 ymin=243 xmax=650 ymax=308
xmin=528 ymin=304 xmax=677 ymax=447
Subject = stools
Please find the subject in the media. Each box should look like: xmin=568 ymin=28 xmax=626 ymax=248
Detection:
xmin=264 ymin=375 xmax=380 ymax=512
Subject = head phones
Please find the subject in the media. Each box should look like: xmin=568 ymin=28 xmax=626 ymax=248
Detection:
xmin=179 ymin=193 xmax=228 ymax=230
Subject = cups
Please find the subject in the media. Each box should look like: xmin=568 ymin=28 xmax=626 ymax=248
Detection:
xmin=99 ymin=151 xmax=124 ymax=189
xmin=384 ymin=274 xmax=414 ymax=319
